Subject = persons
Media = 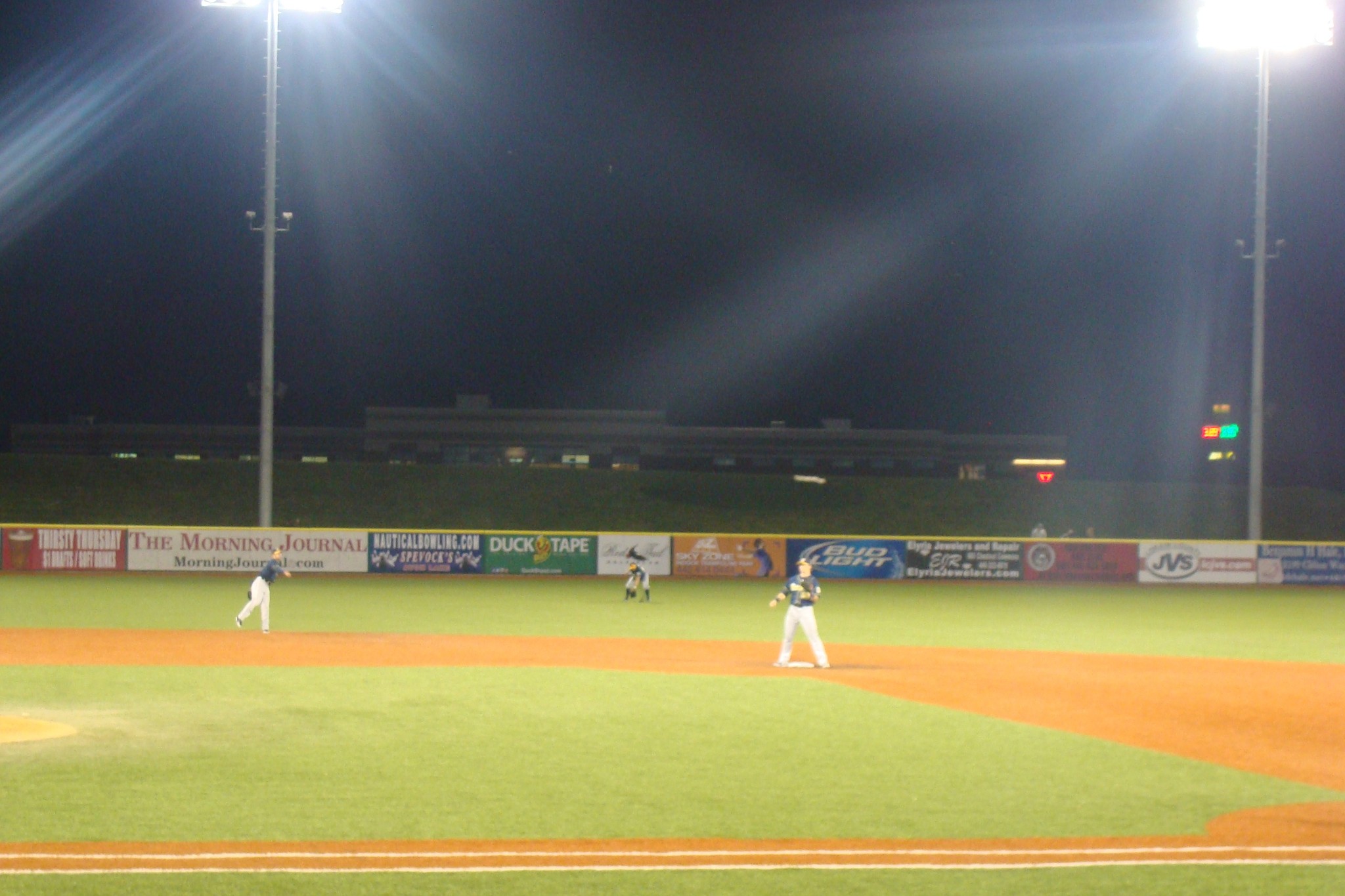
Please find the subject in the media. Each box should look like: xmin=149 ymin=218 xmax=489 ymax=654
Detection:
xmin=1060 ymin=528 xmax=1078 ymax=538
xmin=623 ymin=562 xmax=652 ymax=603
xmin=769 ymin=557 xmax=831 ymax=668
xmin=1031 ymin=522 xmax=1047 ymax=538
xmin=235 ymin=548 xmax=292 ymax=634
xmin=1084 ymin=527 xmax=1095 ymax=538
xmin=738 ymin=538 xmax=774 ymax=578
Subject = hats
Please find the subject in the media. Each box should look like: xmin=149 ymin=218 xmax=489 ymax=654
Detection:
xmin=274 ymin=550 xmax=280 ymax=555
xmin=629 ymin=563 xmax=637 ymax=569
xmin=795 ymin=558 xmax=811 ymax=565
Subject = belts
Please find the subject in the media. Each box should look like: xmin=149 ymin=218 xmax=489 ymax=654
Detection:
xmin=261 ymin=576 xmax=270 ymax=586
xmin=792 ymin=603 xmax=804 ymax=607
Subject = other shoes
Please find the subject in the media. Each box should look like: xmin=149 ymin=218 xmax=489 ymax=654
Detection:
xmin=236 ymin=616 xmax=241 ymax=627
xmin=772 ymin=661 xmax=788 ymax=667
xmin=822 ymin=663 xmax=830 ymax=668
xmin=264 ymin=630 xmax=269 ymax=634
